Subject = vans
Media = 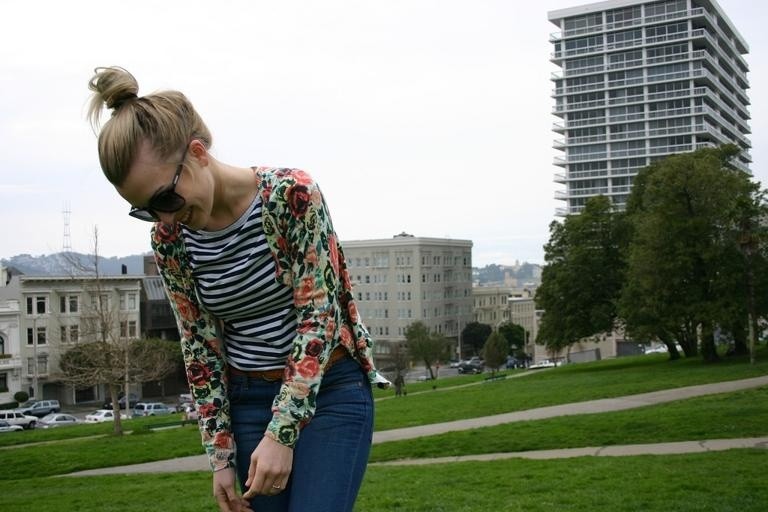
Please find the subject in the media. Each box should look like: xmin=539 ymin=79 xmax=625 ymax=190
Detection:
xmin=0 ymin=410 xmax=39 ymax=430
xmin=13 ymin=398 xmax=61 ymax=415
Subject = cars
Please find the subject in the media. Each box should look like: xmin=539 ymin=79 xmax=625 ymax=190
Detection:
xmin=0 ymin=420 xmax=24 ymax=432
xmin=104 ymin=391 xmax=141 ymax=408
xmin=85 ymin=408 xmax=132 ymax=423
xmin=449 ymin=356 xmax=562 ymax=374
xmin=40 ymin=413 xmax=82 ymax=425
xmin=646 ymin=344 xmax=683 ymax=354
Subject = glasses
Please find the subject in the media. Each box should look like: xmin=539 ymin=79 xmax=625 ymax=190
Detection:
xmin=129 ymin=143 xmax=190 ymax=222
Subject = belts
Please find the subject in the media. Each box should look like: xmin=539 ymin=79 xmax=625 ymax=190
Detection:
xmin=227 ymin=346 xmax=346 ymax=380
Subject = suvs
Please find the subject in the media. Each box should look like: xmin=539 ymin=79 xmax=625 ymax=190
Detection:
xmin=133 ymin=403 xmax=177 ymax=417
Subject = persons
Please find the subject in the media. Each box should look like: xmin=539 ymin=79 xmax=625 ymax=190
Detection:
xmin=182 ymin=413 xmax=186 ymax=427
xmin=88 ymin=67 xmax=393 ymax=512
xmin=394 ymin=373 xmax=407 ymax=397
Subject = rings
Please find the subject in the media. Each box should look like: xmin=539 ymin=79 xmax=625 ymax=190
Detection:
xmin=273 ymin=485 xmax=282 ymax=491
